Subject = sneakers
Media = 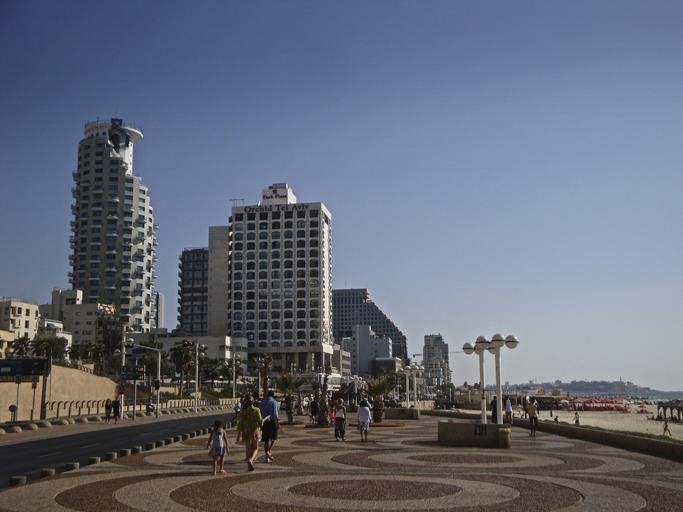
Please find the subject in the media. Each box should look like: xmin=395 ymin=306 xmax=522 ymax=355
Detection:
xmin=247 ymin=458 xmax=254 ymax=471
xmin=266 ymin=450 xmax=274 ymax=463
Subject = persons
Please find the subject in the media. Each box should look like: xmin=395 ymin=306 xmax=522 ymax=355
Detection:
xmin=207 ymin=390 xmax=372 ymax=475
xmin=490 ymin=395 xmax=539 ymax=437
xmin=149 ymin=396 xmax=155 ymax=412
xmin=112 ymin=396 xmax=122 ymax=424
xmin=555 ymin=416 xmax=558 ymax=421
xmin=105 ymin=399 xmax=113 ymax=424
xmin=572 ymin=412 xmax=579 ymax=424
xmin=663 ymin=420 xmax=672 ymax=436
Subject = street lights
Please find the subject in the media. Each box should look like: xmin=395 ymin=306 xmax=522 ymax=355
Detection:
xmin=461 ymin=332 xmax=521 ymax=425
xmin=193 ymin=340 xmax=298 ymax=413
xmin=114 ymin=322 xmax=195 ymax=423
xmin=344 ymin=375 xmax=370 ymax=406
xmin=399 ymin=365 xmax=425 ymax=410
